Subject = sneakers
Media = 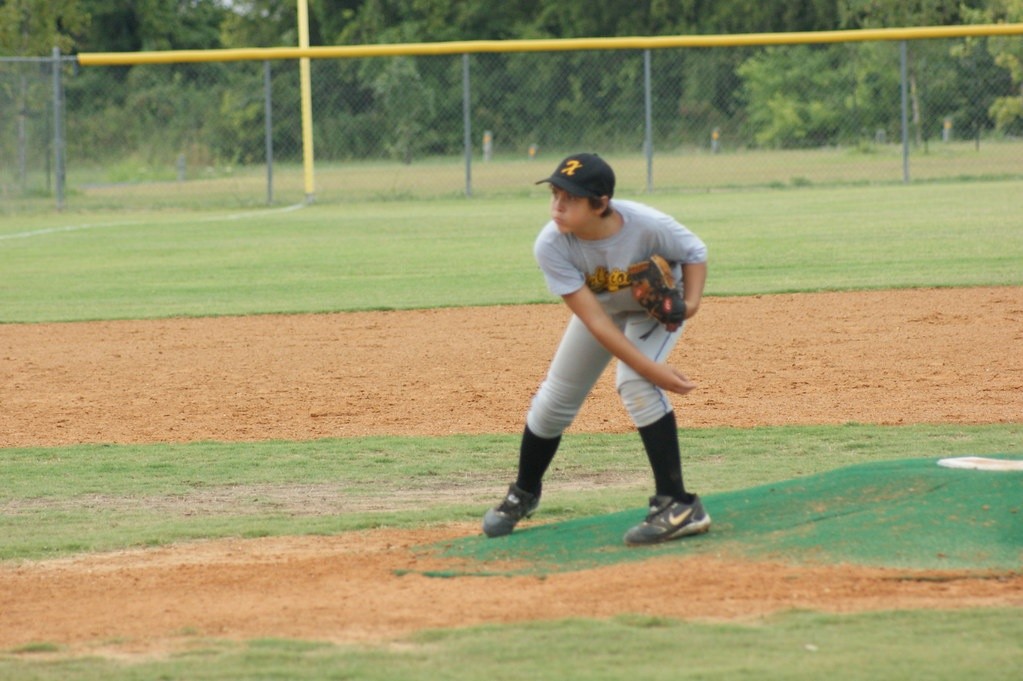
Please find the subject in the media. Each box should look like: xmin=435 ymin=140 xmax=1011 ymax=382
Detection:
xmin=483 ymin=481 xmax=539 ymax=538
xmin=623 ymin=492 xmax=711 ymax=546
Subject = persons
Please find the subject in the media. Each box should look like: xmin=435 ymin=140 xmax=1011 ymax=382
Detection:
xmin=482 ymin=152 xmax=711 ymax=547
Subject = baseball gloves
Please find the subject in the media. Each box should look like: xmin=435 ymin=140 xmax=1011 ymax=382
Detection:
xmin=627 ymin=254 xmax=686 ymax=328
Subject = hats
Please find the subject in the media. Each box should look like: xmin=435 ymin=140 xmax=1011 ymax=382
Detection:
xmin=536 ymin=153 xmax=616 ymax=198
